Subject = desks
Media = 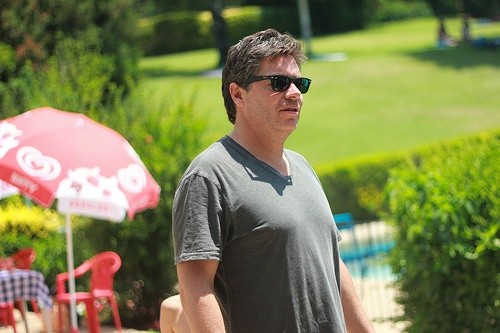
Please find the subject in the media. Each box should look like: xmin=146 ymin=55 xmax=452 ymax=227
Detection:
xmin=0 ymin=269 xmax=54 ymax=333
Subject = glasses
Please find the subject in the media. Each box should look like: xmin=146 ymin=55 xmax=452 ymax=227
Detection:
xmin=238 ymin=75 xmax=311 ymax=94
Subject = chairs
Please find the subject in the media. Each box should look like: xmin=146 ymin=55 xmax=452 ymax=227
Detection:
xmin=55 ymin=251 xmax=122 ymax=333
xmin=0 ymin=302 xmax=17 ymax=333
xmin=0 ymin=248 xmax=41 ymax=320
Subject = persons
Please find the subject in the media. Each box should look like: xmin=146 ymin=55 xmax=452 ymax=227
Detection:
xmin=159 ymin=286 xmax=191 ymax=333
xmin=436 ymin=29 xmax=462 ymax=47
xmin=170 ymin=29 xmax=376 ymax=333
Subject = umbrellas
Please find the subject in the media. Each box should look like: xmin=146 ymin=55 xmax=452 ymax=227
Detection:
xmin=0 ymin=106 xmax=162 ymax=333
